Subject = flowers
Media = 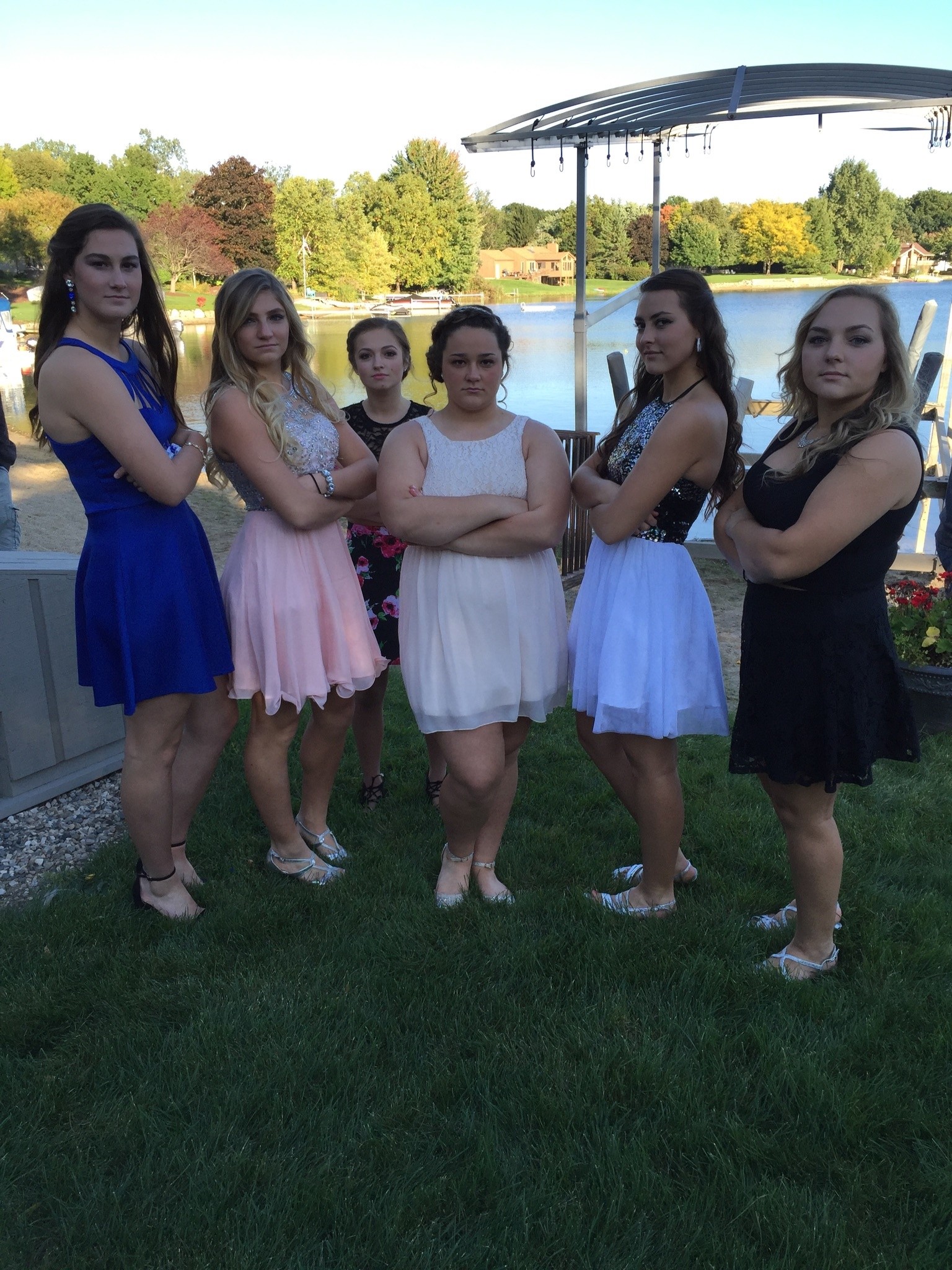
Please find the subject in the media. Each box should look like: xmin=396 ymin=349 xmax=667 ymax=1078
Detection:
xmin=884 ymin=571 xmax=952 ymax=673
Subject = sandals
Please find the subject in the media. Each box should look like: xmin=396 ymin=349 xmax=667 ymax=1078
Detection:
xmin=470 ymin=860 xmax=516 ymax=907
xmin=292 ymin=814 xmax=348 ymax=869
xmin=613 ymin=859 xmax=698 ymax=884
xmin=435 ymin=841 xmax=476 ymax=911
xmin=424 ymin=769 xmax=452 ymax=814
xmin=265 ymin=842 xmax=346 ymax=888
xmin=166 ymin=838 xmax=187 ymax=850
xmin=360 ymin=772 xmax=389 ymax=813
xmin=133 ymin=862 xmax=209 ymax=927
xmin=750 ymin=893 xmax=843 ymax=936
xmin=584 ymin=887 xmax=676 ymax=922
xmin=753 ymin=944 xmax=839 ymax=980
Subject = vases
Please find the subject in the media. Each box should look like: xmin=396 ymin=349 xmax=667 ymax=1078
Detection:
xmin=895 ymin=660 xmax=952 ymax=698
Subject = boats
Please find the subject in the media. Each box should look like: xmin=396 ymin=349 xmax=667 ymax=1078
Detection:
xmin=368 ymin=291 xmax=454 ymax=315
xmin=520 ymin=302 xmax=556 ymax=313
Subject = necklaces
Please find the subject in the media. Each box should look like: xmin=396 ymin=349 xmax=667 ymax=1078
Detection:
xmin=796 ymin=422 xmax=831 ymax=450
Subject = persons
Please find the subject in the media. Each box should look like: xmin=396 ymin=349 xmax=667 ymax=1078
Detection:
xmin=934 ymin=467 xmax=952 ymax=576
xmin=712 ymin=284 xmax=932 ymax=990
xmin=200 ymin=267 xmax=381 ymax=892
xmin=373 ymin=300 xmax=576 ymax=912
xmin=341 ymin=314 xmax=448 ymax=820
xmin=561 ymin=268 xmax=748 ymax=924
xmin=0 ymin=392 xmax=22 ymax=551
xmin=30 ymin=199 xmax=237 ymax=921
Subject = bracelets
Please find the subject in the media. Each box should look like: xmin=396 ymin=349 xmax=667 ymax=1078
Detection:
xmin=307 ymin=472 xmax=322 ymax=496
xmin=183 ymin=441 xmax=207 ymax=465
xmin=320 ymin=468 xmax=335 ymax=499
xmin=742 ymin=569 xmax=747 ymax=582
